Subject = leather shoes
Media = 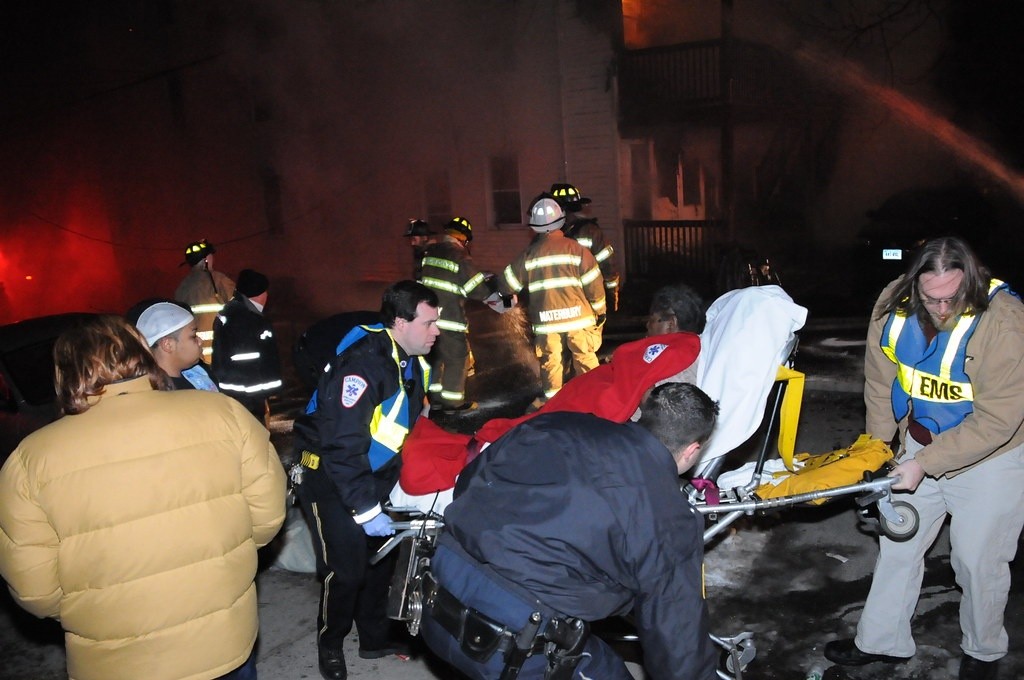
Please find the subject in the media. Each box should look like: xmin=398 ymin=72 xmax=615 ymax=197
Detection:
xmin=318 ymin=643 xmax=346 ymax=680
xmin=959 ymin=654 xmax=999 ymax=680
xmin=825 ymin=639 xmax=911 ymax=665
xmin=358 ymin=639 xmax=416 ymax=659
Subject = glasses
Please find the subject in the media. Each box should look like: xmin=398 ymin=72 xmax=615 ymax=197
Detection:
xmin=918 ymin=288 xmax=959 ymax=306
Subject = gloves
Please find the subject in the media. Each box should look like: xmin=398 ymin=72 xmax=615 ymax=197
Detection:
xmin=363 ymin=513 xmax=396 ymax=537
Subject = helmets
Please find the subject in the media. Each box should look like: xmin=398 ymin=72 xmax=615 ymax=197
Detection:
xmin=445 ymin=216 xmax=473 ymax=241
xmin=549 ymin=183 xmax=592 ymax=209
xmin=182 ymin=241 xmax=215 ymax=266
xmin=529 ymin=198 xmax=565 ymax=233
xmin=403 ymin=218 xmax=437 ymax=236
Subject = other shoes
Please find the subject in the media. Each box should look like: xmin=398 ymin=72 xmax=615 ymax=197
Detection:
xmin=431 ymin=402 xmax=441 ymax=410
xmin=445 ymin=402 xmax=478 ymax=414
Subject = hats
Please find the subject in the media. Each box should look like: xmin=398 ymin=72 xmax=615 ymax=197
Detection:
xmin=237 ymin=269 xmax=269 ymax=297
xmin=137 ymin=302 xmax=194 ymax=347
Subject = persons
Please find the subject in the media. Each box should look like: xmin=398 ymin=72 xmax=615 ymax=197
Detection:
xmin=136 ymin=301 xmax=204 ymax=390
xmin=406 ymin=219 xmax=475 ymax=376
xmin=823 ymin=238 xmax=1024 ymax=679
xmin=212 ymin=270 xmax=289 ymax=430
xmin=293 ymin=282 xmax=440 ymax=677
xmin=420 ymin=216 xmax=499 ymax=412
xmin=625 ymin=284 xmax=705 ymax=422
xmin=174 ymin=240 xmax=236 ymax=363
xmin=0 ymin=314 xmax=288 ymax=679
xmin=496 ymin=198 xmax=607 ymax=396
xmin=420 ymin=383 xmax=720 ymax=680
xmin=530 ymin=184 xmax=621 ymax=376
